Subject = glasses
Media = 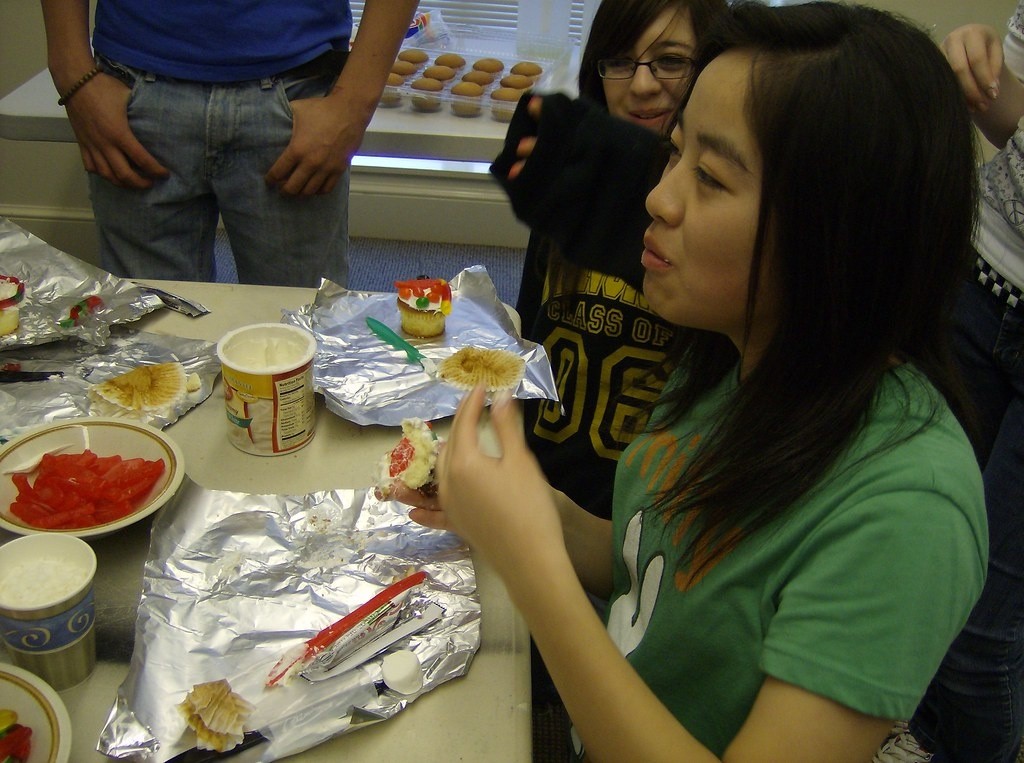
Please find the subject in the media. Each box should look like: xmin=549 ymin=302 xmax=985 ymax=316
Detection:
xmin=599 ymin=55 xmax=695 ymax=80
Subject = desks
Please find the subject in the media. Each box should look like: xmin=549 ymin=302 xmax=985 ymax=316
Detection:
xmin=0 ymin=278 xmax=536 ymax=763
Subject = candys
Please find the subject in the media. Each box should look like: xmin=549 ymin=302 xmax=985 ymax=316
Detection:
xmin=0 ymin=709 xmax=33 ymax=763
xmin=8 ymin=449 xmax=166 ymax=528
xmin=59 ymin=296 xmax=102 ymax=327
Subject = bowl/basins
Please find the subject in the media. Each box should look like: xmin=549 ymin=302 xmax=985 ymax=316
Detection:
xmin=1 ymin=662 xmax=73 ymax=763
xmin=0 ymin=418 xmax=185 ymax=538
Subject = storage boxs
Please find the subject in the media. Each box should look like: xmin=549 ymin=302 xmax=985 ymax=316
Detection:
xmin=379 ymin=34 xmax=575 ymax=120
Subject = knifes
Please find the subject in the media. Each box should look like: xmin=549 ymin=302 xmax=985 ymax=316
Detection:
xmin=1 ymin=370 xmax=64 ymax=383
xmin=164 ymin=679 xmax=386 ymax=763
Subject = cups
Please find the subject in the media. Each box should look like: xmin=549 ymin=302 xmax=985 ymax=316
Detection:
xmin=1 ymin=532 xmax=98 ymax=693
xmin=217 ymin=323 xmax=316 ymax=456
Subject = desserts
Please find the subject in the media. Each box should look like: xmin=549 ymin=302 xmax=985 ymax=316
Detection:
xmin=393 ymin=277 xmax=452 ymax=337
xmin=379 ymin=48 xmax=544 ymax=121
xmin=379 ymin=417 xmax=445 ymax=494
xmin=0 ymin=274 xmax=24 ymax=336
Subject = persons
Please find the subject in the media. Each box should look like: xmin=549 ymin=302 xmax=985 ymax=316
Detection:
xmin=40 ymin=0 xmax=416 ymax=288
xmin=374 ymin=0 xmax=990 ymax=763
xmin=489 ymin=0 xmax=1024 ymax=763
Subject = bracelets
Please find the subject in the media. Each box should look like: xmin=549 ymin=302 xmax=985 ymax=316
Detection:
xmin=58 ymin=64 xmax=104 ymax=106
